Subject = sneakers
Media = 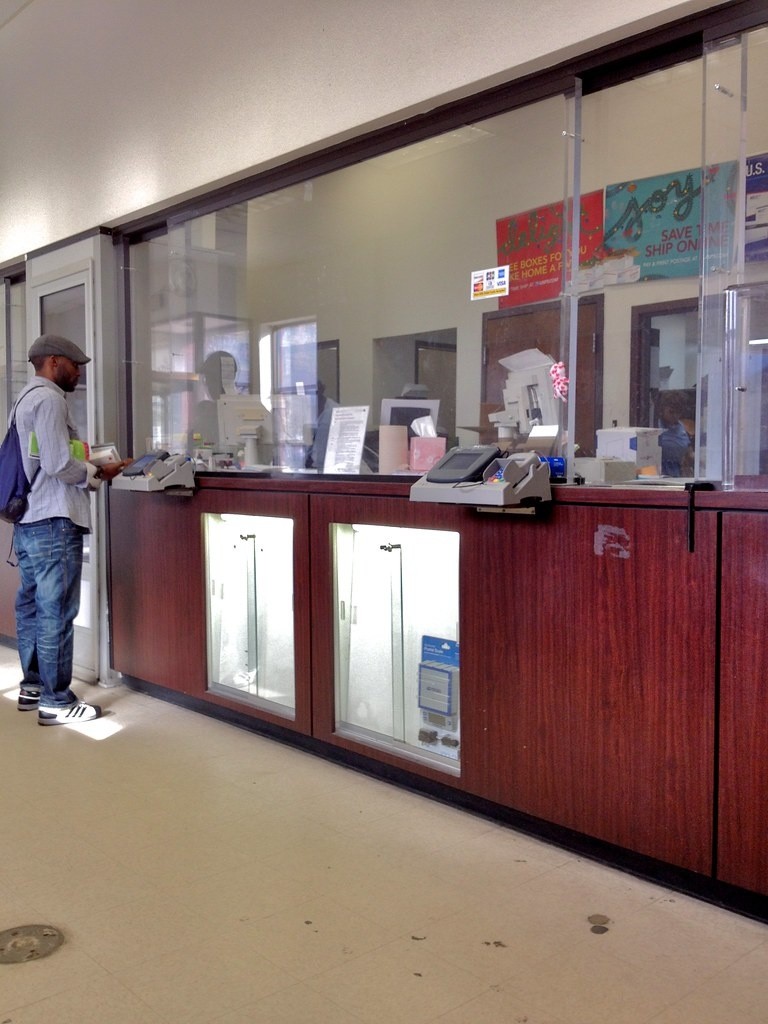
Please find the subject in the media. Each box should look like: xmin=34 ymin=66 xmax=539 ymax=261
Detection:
xmin=37 ymin=700 xmax=101 ymax=726
xmin=17 ymin=686 xmax=41 ymax=711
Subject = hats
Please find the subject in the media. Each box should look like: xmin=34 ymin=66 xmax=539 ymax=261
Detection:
xmin=28 ymin=333 xmax=91 ymax=365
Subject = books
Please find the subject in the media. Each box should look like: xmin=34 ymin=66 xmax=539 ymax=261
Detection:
xmin=91 ymin=442 xmax=124 ymax=469
xmin=28 ymin=431 xmax=89 ymax=463
xmin=88 ymin=448 xmax=124 ymax=470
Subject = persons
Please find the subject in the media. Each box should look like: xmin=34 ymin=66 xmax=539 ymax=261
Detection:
xmin=0 ymin=334 xmax=125 ymax=726
xmin=203 ymin=353 xmax=274 ymax=465
xmin=292 ymin=379 xmax=340 ymax=468
xmin=657 ymin=390 xmax=690 ymax=478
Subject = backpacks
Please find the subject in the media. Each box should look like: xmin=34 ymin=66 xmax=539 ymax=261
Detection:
xmin=0 ymin=385 xmax=68 ymax=523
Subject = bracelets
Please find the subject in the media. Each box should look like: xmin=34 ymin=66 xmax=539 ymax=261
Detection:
xmin=94 ymin=466 xmax=104 ymax=479
